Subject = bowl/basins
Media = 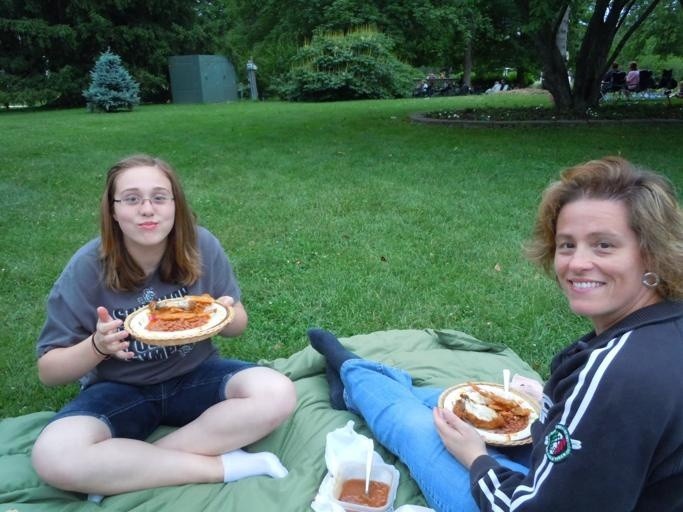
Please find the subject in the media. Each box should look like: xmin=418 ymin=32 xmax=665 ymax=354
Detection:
xmin=328 ymin=461 xmax=399 ymax=510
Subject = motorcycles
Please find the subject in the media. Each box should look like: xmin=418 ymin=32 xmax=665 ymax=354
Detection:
xmin=412 ymin=72 xmax=469 ymax=97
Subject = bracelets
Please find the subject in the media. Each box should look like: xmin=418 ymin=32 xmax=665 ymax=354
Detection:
xmin=92 ymin=331 xmax=110 ymax=359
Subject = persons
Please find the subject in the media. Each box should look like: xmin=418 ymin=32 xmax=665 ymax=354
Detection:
xmin=31 ymin=156 xmax=297 ymax=504
xmin=306 ymin=158 xmax=683 ymax=512
xmin=422 ymin=72 xmax=448 ymax=96
xmin=675 ymin=78 xmax=683 ymax=97
xmin=485 ymin=79 xmax=505 ymax=95
xmin=605 ymin=60 xmax=640 ymax=90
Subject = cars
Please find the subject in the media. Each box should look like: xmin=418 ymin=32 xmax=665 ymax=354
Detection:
xmin=494 ymin=66 xmax=513 ymax=77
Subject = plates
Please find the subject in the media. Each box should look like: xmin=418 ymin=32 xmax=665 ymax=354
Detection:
xmin=122 ymin=295 xmax=233 ymax=346
xmin=438 ymin=381 xmax=544 ymax=445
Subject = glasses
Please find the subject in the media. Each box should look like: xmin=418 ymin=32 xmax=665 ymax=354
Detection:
xmin=112 ymin=193 xmax=175 ymax=206
